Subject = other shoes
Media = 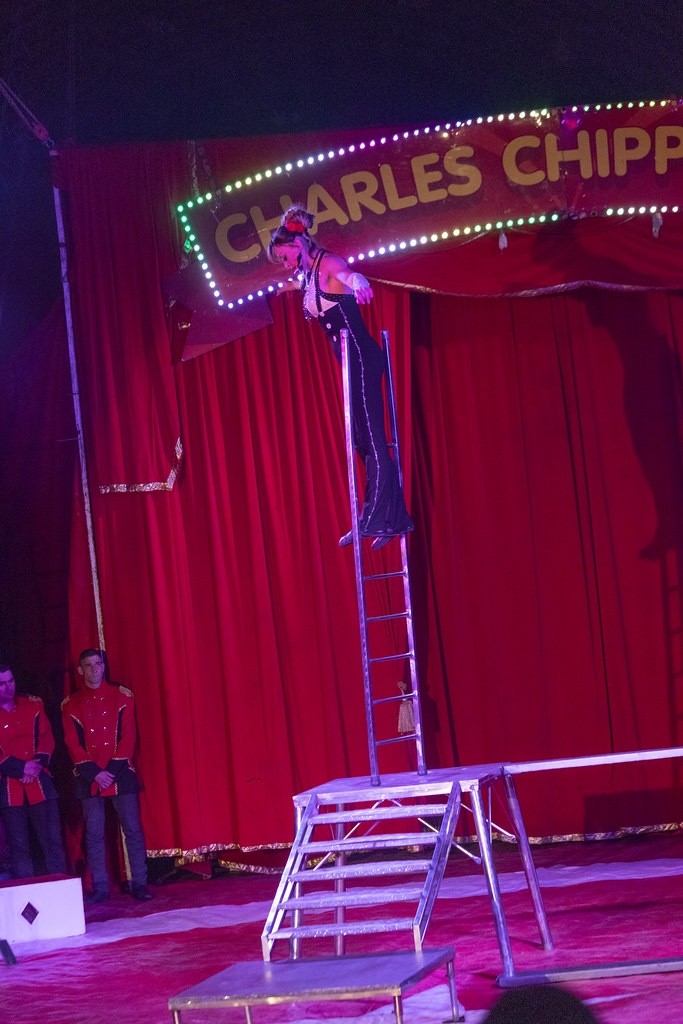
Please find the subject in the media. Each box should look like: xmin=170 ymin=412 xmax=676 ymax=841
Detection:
xmin=85 ymin=895 xmax=110 ymax=903
xmin=338 ymin=523 xmax=392 ymax=546
xmin=132 ymin=886 xmax=153 ymax=901
xmin=371 ymin=521 xmax=413 ymax=551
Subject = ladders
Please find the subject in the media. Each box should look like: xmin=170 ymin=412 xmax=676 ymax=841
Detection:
xmin=341 ymin=330 xmax=429 ymax=785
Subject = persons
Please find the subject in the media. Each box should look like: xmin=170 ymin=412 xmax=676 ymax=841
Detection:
xmin=484 ymin=983 xmax=596 ymax=1024
xmin=0 ymin=649 xmax=154 ymax=904
xmin=268 ymin=206 xmax=414 ymax=547
